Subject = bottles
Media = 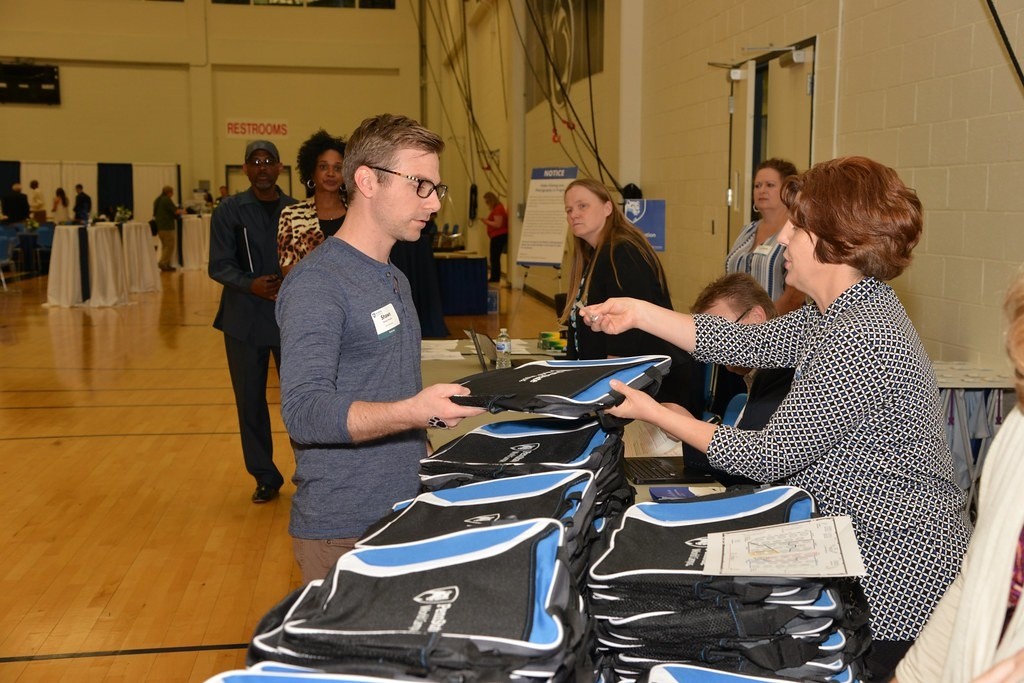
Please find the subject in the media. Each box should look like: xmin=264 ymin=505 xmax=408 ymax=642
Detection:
xmin=494 ymin=328 xmax=511 ymax=369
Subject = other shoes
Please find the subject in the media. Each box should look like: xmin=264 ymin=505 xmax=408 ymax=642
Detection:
xmin=159 ymin=264 xmax=176 ymax=272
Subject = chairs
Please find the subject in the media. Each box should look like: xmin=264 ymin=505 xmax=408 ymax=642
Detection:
xmin=1 ymin=222 xmax=56 ymax=292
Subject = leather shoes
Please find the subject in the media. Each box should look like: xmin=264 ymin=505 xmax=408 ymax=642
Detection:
xmin=252 ymin=476 xmax=283 ymax=502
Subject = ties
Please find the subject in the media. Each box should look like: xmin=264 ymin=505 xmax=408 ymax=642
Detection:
xmin=992 ymin=525 xmax=1023 ymax=651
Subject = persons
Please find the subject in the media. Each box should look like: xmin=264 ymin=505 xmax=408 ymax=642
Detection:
xmin=1 ymin=183 xmax=30 ymax=223
xmin=208 ymin=140 xmax=299 ymax=501
xmin=275 ymin=114 xmax=486 ymax=581
xmin=658 ymin=272 xmax=794 ymax=485
xmin=580 ymin=157 xmax=971 ymax=683
xmin=52 ymin=188 xmax=71 ymax=224
xmin=890 ymin=271 xmax=1024 ymax=683
xmin=558 ymin=179 xmax=674 ymax=360
xmin=277 ymin=130 xmax=348 ymax=277
xmin=217 ymin=186 xmax=230 ymax=200
xmin=72 ymin=185 xmax=91 ymax=220
xmin=713 ymin=159 xmax=809 ymax=417
xmin=480 ymin=192 xmax=508 ymax=282
xmin=421 ymin=213 xmax=438 ymax=246
xmin=153 ymin=186 xmax=187 ymax=271
xmin=203 ymin=190 xmax=213 ymax=202
xmin=28 ymin=181 xmax=46 ymax=224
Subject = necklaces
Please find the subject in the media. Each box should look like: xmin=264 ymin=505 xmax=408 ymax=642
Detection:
xmin=318 ymin=208 xmax=340 ymax=220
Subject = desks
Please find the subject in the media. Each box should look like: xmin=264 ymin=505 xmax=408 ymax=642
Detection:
xmin=41 ymin=222 xmax=162 ymax=307
xmin=181 ymin=213 xmax=212 ymax=271
xmin=419 ymin=337 xmax=683 ymax=457
xmin=434 ymin=252 xmax=488 ymax=317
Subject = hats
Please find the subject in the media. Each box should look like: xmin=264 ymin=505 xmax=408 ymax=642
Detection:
xmin=245 ymin=140 xmax=279 ymax=163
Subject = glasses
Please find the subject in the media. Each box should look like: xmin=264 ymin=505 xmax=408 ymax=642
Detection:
xmin=248 ymin=157 xmax=274 ymax=166
xmin=368 ymin=164 xmax=447 ymax=201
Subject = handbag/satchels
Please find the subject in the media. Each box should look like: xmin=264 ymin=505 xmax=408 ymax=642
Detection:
xmin=445 ymin=354 xmax=670 ymax=419
xmin=586 ymin=483 xmax=872 ymax=683
xmin=149 ymin=220 xmax=158 ymax=236
xmin=206 ymin=417 xmax=635 ymax=683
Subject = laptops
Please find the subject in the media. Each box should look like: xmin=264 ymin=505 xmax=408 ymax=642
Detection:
xmin=618 ymin=453 xmax=715 ymax=485
